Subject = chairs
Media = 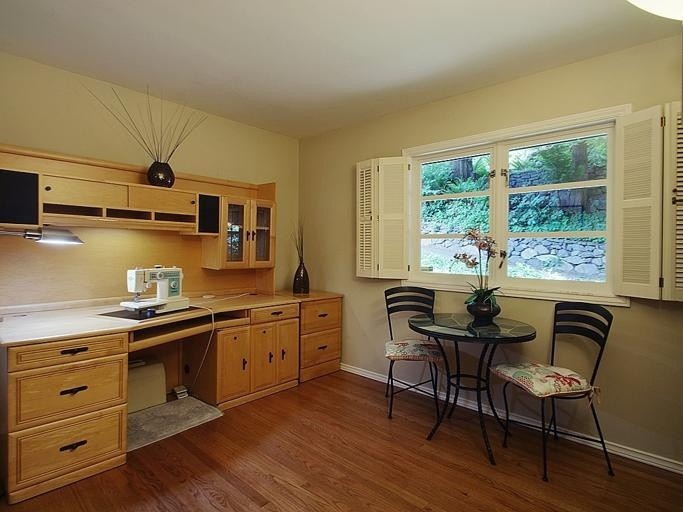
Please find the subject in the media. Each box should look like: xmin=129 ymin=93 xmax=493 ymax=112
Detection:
xmin=501 ymin=301 xmax=616 ymax=483
xmin=380 ymin=285 xmax=448 ymax=420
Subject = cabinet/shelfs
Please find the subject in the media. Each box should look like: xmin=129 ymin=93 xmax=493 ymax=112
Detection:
xmin=203 ymin=194 xmax=278 ymax=270
xmin=179 ymin=304 xmax=299 ymax=411
xmin=299 ymin=296 xmax=344 ymax=385
xmin=0 ymin=333 xmax=130 ymax=506
xmin=38 ymin=172 xmax=198 ymax=245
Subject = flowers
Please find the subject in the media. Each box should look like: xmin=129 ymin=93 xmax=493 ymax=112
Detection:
xmin=446 ymin=226 xmax=509 ymax=314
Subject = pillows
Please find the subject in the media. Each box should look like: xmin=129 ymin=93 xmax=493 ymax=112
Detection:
xmin=489 ymin=360 xmax=591 ymax=400
xmin=384 ymin=339 xmax=445 ymax=362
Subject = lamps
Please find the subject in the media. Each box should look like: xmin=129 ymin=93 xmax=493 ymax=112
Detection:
xmin=0 ymin=227 xmax=86 ymax=245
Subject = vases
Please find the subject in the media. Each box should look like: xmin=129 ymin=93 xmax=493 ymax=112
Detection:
xmin=467 ymin=298 xmax=503 ymax=330
xmin=291 ymin=261 xmax=310 ymax=297
xmin=147 ymin=161 xmax=176 ymax=188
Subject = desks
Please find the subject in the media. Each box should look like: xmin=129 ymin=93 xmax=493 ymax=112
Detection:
xmin=407 ymin=313 xmax=536 ymax=466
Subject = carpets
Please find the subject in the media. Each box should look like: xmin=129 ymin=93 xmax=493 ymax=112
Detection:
xmin=124 ymin=395 xmax=223 ymax=455
